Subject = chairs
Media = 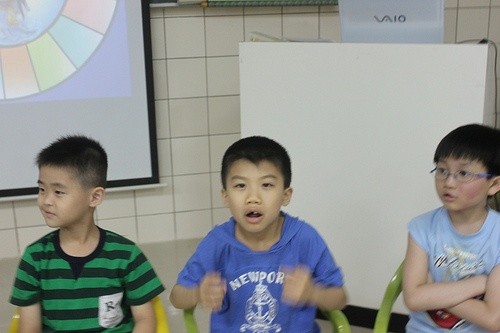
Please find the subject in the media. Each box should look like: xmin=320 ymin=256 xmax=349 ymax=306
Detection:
xmin=183 ymin=296 xmax=352 ymax=333
xmin=8 ymin=290 xmax=169 ymax=333
xmin=372 ymin=254 xmax=405 ymax=333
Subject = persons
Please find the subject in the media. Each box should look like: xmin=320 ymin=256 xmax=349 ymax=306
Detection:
xmin=170 ymin=136 xmax=347 ymax=333
xmin=9 ymin=136 xmax=165 ymax=333
xmin=403 ymin=123 xmax=500 ymax=333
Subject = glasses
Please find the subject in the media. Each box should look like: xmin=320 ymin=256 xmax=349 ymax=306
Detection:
xmin=430 ymin=167 xmax=492 ymax=183
